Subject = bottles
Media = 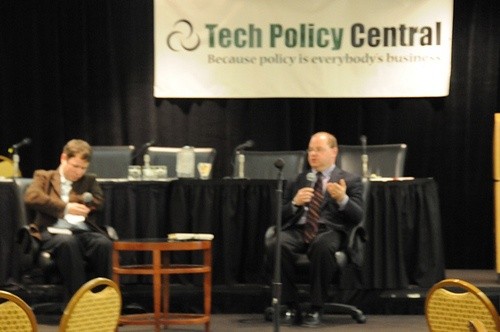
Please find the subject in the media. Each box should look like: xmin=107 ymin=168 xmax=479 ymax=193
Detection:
xmin=12 ymin=154 xmax=21 ymax=178
xmin=175 ymin=146 xmax=195 ymax=178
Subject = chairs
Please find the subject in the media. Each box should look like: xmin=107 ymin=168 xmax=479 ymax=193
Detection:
xmin=0 ymin=177 xmax=121 ymax=332
xmin=145 ymin=146 xmax=216 ymax=179
xmin=86 ymin=145 xmax=131 ymax=180
xmin=425 ymin=278 xmax=500 ymax=332
xmin=233 ymin=143 xmax=408 ymax=180
xmin=259 ymin=178 xmax=371 ymax=323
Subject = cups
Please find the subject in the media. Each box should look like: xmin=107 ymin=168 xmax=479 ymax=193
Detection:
xmin=142 ymin=166 xmax=156 ymax=181
xmin=127 ymin=164 xmax=142 ymax=181
xmin=156 ymin=165 xmax=169 ymax=181
xmin=197 ymin=162 xmax=212 ymax=178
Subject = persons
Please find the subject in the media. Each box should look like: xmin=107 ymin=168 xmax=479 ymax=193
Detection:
xmin=266 ymin=132 xmax=368 ymax=328
xmin=24 ymin=140 xmax=114 ymax=314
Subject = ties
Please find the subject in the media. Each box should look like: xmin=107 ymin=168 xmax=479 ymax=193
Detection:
xmin=304 ymin=171 xmax=323 ymax=244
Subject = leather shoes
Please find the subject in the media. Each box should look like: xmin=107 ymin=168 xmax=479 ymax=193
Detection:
xmin=300 ymin=310 xmax=321 ymax=328
xmin=280 ymin=310 xmax=301 ymax=327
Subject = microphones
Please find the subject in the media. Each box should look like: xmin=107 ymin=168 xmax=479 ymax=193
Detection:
xmin=8 ymin=138 xmax=31 ymax=152
xmin=135 ymin=135 xmax=170 ymax=165
xmin=360 ymin=135 xmax=368 ymax=155
xmin=235 ymin=139 xmax=255 ymax=153
xmin=304 ymin=171 xmax=318 ymax=211
xmin=83 ymin=190 xmax=93 ymax=218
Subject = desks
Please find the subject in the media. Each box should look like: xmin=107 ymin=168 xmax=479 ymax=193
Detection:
xmin=0 ymin=176 xmax=444 ymax=309
xmin=111 ymin=239 xmax=211 ymax=332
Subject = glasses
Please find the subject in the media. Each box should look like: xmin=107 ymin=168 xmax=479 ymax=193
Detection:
xmin=306 ymin=145 xmax=337 ymax=153
